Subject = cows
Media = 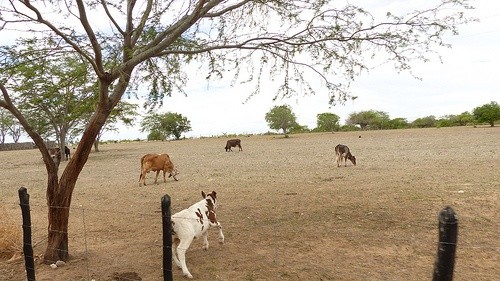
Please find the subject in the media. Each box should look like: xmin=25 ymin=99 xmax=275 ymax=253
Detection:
xmin=138 ymin=153 xmax=180 ymax=188
xmin=335 ymin=144 xmax=356 ymax=168
xmin=224 ymin=138 xmax=242 ymax=152
xmin=41 ymin=146 xmax=72 ymax=163
xmin=170 ymin=190 xmax=225 ymax=279
